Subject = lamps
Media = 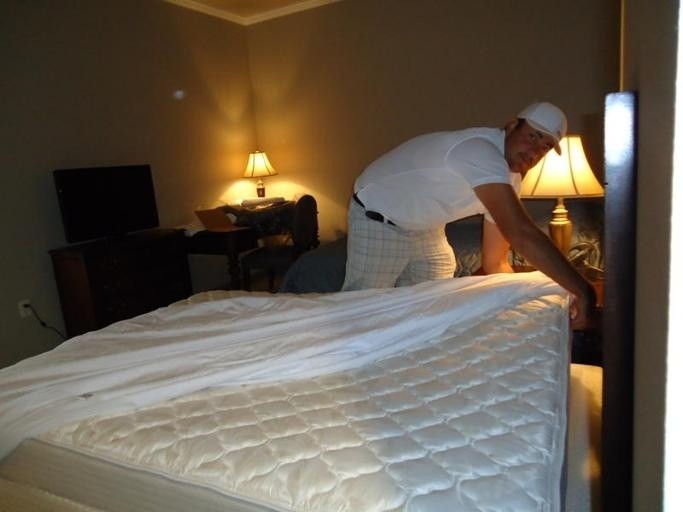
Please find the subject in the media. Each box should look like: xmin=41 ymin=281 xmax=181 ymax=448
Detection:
xmin=242 ymin=150 xmax=277 ymax=197
xmin=519 ymin=134 xmax=604 ymax=257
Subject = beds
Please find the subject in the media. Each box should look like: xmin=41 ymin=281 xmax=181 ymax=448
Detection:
xmin=0 ymin=269 xmax=603 ymax=511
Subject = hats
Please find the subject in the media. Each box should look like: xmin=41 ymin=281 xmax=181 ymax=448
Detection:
xmin=516 ymin=101 xmax=568 ymax=156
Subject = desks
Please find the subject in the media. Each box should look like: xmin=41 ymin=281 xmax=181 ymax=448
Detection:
xmin=175 ymin=224 xmax=258 ymax=289
xmin=472 ymin=264 xmax=604 ymax=308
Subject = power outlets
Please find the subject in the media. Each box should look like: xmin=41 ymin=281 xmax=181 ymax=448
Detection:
xmin=18 ymin=299 xmax=31 ymax=318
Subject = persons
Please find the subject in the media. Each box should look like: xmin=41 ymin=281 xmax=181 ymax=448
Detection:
xmin=340 ymin=102 xmax=597 ymax=331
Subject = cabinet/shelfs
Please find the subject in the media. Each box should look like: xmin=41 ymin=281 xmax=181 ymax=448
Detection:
xmin=49 ymin=227 xmax=194 ymax=339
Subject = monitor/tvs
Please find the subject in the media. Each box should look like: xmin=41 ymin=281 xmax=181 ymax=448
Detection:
xmin=53 ymin=164 xmax=160 ymax=243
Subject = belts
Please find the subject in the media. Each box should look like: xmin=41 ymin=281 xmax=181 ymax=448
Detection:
xmin=353 ymin=193 xmax=395 ymax=226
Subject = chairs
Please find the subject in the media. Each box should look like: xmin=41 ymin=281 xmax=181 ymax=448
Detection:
xmin=242 ymin=195 xmax=318 ymax=291
